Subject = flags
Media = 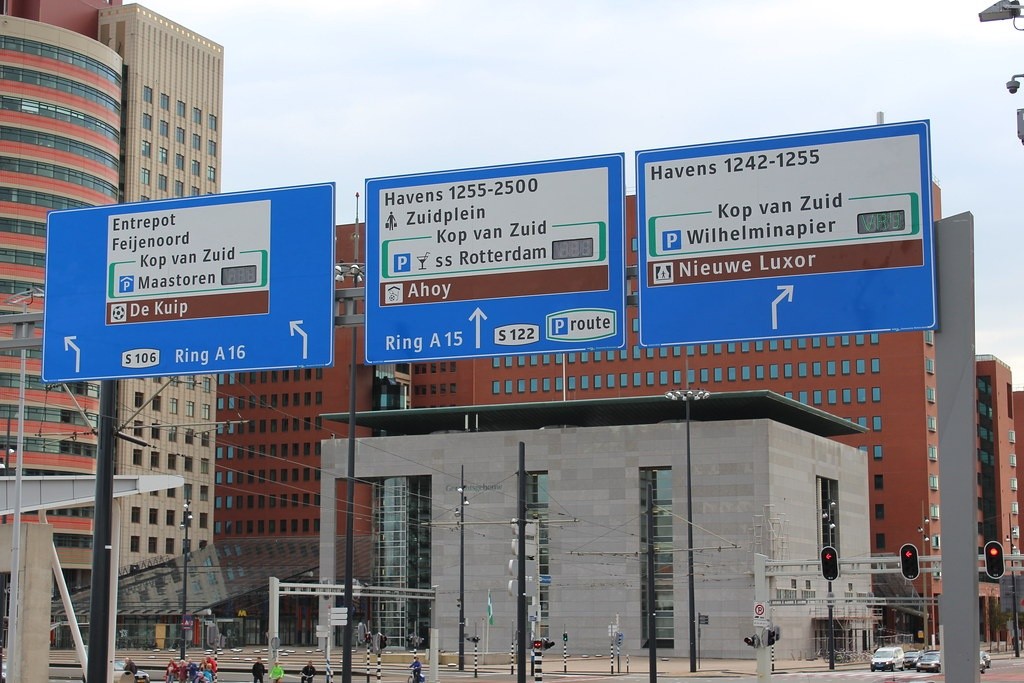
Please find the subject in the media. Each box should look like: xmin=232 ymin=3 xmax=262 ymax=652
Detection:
xmin=487 ymin=590 xmax=494 ymax=625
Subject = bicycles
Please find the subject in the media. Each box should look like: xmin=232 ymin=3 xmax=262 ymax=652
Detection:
xmin=408 ymin=667 xmax=415 ymax=683
xmin=825 ymin=649 xmax=872 ymax=663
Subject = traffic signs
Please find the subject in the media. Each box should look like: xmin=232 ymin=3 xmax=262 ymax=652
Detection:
xmin=634 ymin=118 xmax=937 ymax=349
xmin=41 ymin=181 xmax=334 ymax=382
xmin=364 ymin=152 xmax=626 ymax=364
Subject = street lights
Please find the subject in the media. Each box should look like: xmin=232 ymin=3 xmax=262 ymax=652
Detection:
xmin=918 ymin=516 xmax=930 ymax=651
xmin=665 ymin=390 xmax=712 ymax=673
xmin=822 ymin=502 xmax=837 ymax=668
xmin=335 ymin=263 xmax=364 ymax=681
xmin=453 ymin=485 xmax=473 ymax=670
xmin=180 ymin=501 xmax=193 ymax=660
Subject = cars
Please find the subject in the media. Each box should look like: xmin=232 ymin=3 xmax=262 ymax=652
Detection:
xmin=81 ymin=662 xmax=150 ymax=683
xmin=870 ymin=646 xmax=991 ymax=676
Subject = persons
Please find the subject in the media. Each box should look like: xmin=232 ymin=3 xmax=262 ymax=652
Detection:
xmin=165 ymin=656 xmax=217 ymax=683
xmin=300 ymin=660 xmax=316 ymax=683
xmin=251 ymin=657 xmax=265 ymax=683
xmin=123 ymin=657 xmax=136 ymax=683
xmin=408 ymin=656 xmax=422 ymax=683
xmin=268 ymin=661 xmax=284 ymax=683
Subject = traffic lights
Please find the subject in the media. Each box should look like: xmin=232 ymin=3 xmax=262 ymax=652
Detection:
xmin=534 ymin=641 xmax=555 ymax=650
xmin=743 ymin=630 xmax=775 ymax=646
xmin=984 ymin=541 xmax=1005 ymax=579
xmin=819 ymin=546 xmax=839 ymax=581
xmin=364 ymin=632 xmax=387 ymax=649
xmin=562 ymin=634 xmax=567 ymax=641
xmin=899 ymin=544 xmax=920 ymax=580
